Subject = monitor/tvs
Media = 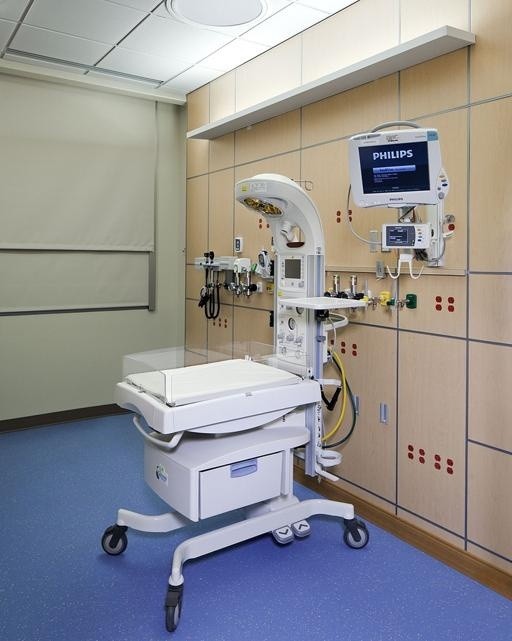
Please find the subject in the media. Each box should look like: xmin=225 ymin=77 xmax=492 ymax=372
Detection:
xmin=347 ymin=129 xmax=451 ymax=208
